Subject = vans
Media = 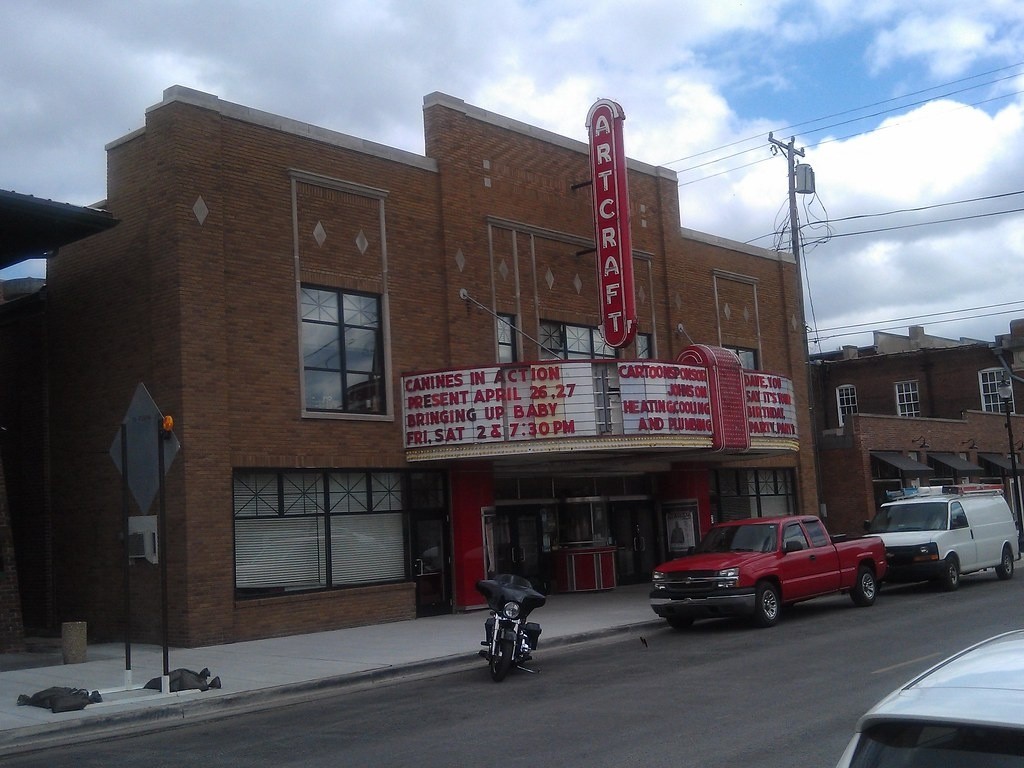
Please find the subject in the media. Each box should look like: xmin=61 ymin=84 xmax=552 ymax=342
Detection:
xmin=862 ymin=483 xmax=1021 ymax=592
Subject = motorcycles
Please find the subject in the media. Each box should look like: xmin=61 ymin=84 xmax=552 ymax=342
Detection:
xmin=475 ymin=573 xmax=546 ymax=683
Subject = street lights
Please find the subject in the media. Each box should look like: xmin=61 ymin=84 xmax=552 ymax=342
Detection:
xmin=998 ymin=374 xmax=1024 ymax=552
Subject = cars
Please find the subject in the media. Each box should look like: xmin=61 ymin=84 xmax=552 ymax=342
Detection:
xmin=835 ymin=628 xmax=1023 ymax=768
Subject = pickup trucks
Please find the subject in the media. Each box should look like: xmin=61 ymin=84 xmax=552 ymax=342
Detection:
xmin=648 ymin=514 xmax=888 ymax=630
